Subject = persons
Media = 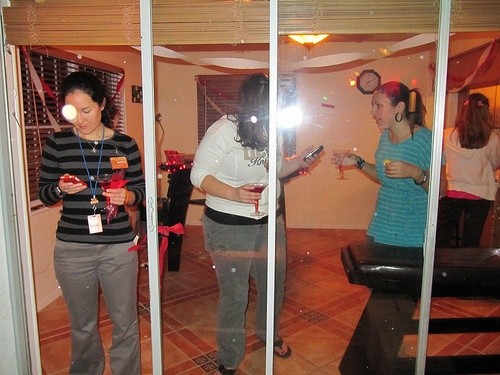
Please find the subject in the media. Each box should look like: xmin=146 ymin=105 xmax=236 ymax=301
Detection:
xmin=38 ymin=70 xmax=145 ymax=375
xmin=435 ymin=92 xmax=500 ymax=248
xmin=331 ymin=81 xmax=448 ymax=246
xmin=190 ymin=74 xmax=320 ymax=375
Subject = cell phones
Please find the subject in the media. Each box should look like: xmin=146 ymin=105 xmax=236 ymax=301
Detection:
xmin=60 ymin=175 xmax=88 ymax=187
xmin=304 ymin=145 xmax=323 ymax=163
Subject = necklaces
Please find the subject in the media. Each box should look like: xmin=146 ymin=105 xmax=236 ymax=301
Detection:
xmin=80 ymin=130 xmax=101 ymax=153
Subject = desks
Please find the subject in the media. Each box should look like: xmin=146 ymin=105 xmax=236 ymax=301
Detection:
xmin=159 ymin=164 xmax=302 ymax=271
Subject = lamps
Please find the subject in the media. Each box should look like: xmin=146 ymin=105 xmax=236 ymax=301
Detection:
xmin=288 ymin=35 xmax=330 ymax=51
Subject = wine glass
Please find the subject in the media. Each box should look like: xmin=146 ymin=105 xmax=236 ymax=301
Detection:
xmin=245 ymin=183 xmax=268 ymax=216
xmin=333 ymin=149 xmax=351 ymax=180
xmin=91 ymin=174 xmax=118 ymax=212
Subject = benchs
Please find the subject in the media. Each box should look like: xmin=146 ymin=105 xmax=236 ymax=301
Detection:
xmin=337 ymin=243 xmax=500 ymax=375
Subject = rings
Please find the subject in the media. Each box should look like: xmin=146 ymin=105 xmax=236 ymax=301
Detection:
xmin=63 ymin=186 xmax=69 ymax=189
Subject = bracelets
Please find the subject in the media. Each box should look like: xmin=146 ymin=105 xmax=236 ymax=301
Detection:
xmin=298 ymin=157 xmax=307 ymax=171
xmin=356 ymin=157 xmax=365 ymax=169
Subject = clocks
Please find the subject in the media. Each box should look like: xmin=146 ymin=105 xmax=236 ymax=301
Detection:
xmin=356 ymin=69 xmax=381 ymax=94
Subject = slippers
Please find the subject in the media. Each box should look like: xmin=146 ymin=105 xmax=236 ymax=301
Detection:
xmin=213 ymin=365 xmax=236 ymax=375
xmin=259 ymin=336 xmax=292 ymax=359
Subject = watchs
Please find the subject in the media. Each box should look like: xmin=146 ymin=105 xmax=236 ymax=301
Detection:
xmin=414 ymin=171 xmax=427 ymax=185
xmin=53 ymin=183 xmax=68 ymax=196
